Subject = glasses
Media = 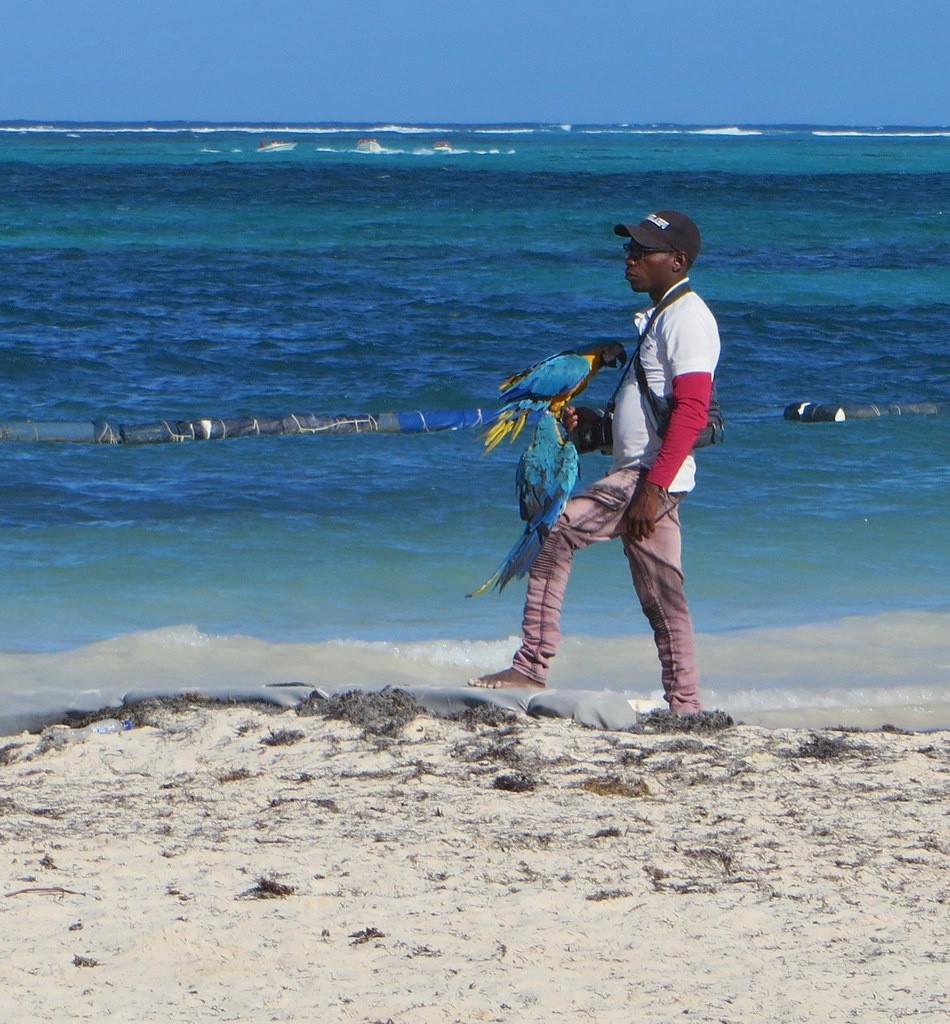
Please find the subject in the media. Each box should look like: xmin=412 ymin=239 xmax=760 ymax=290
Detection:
xmin=623 ymin=244 xmax=674 ymax=260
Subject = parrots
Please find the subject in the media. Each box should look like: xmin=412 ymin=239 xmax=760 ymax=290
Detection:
xmin=471 ymin=334 xmax=629 ymax=459
xmin=464 ymin=410 xmax=584 ymax=602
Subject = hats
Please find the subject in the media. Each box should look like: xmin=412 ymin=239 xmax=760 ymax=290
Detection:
xmin=613 ymin=209 xmax=701 ymax=264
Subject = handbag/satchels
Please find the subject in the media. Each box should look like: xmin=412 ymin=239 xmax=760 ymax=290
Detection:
xmin=631 ymin=281 xmax=725 ymax=449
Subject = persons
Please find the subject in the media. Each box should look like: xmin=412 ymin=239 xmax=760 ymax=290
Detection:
xmin=466 ymin=209 xmax=720 ymax=723
